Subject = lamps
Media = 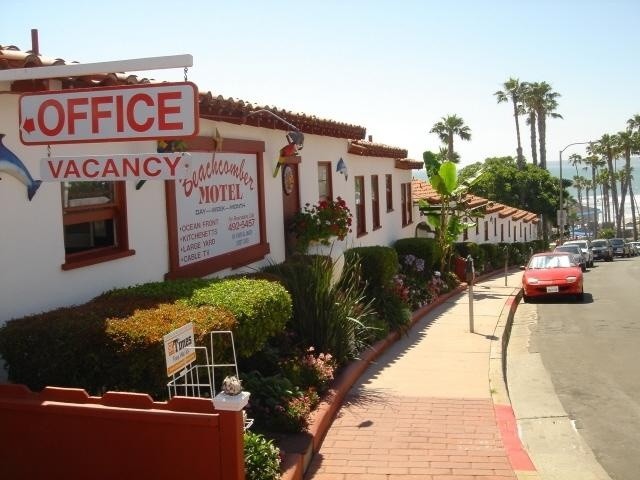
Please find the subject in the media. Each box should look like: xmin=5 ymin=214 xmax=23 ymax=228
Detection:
xmin=246 ymin=108 xmax=304 ymax=150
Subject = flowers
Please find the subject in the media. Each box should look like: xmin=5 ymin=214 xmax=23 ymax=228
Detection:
xmin=221 ymin=375 xmax=242 ymax=396
xmin=252 ymin=378 xmax=321 ymax=436
xmin=286 ymin=197 xmax=353 ymax=241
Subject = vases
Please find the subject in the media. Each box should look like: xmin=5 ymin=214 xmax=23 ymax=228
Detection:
xmin=263 ymin=417 xmax=296 ymax=436
xmin=305 ymin=235 xmax=345 ymax=297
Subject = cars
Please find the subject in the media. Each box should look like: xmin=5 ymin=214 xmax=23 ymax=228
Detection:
xmin=554 ymin=239 xmax=640 ymax=271
xmin=522 ymin=252 xmax=584 ymax=303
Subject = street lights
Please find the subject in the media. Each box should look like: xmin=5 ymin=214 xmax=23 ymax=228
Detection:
xmin=560 ymin=141 xmax=591 ymax=239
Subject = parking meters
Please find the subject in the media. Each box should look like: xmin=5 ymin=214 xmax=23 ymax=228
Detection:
xmin=529 ymin=247 xmax=533 ymax=256
xmin=465 ymin=255 xmax=475 ymax=334
xmin=503 ymin=245 xmax=508 ymax=285
